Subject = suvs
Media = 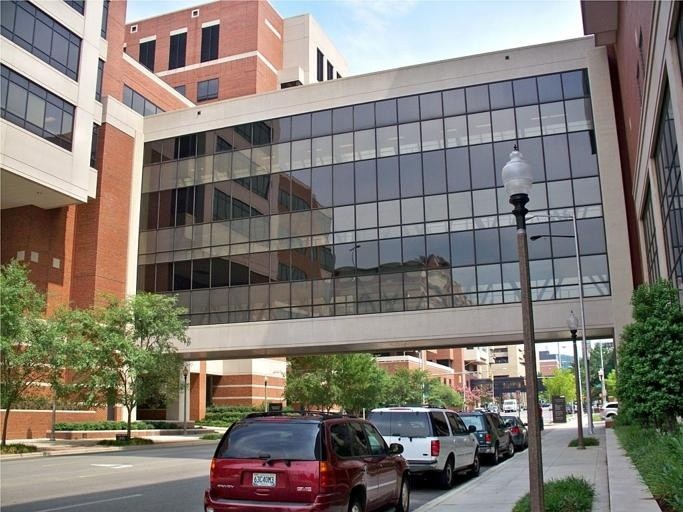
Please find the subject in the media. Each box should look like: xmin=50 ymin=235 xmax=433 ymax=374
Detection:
xmin=599 ymin=402 xmax=618 ymax=420
xmin=204 ymin=409 xmax=412 ymax=510
xmin=366 ymin=404 xmax=482 ymax=490
xmin=459 ymin=411 xmax=516 ymax=466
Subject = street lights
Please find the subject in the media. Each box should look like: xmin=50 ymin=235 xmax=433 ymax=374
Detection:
xmin=529 ymin=234 xmax=575 ymax=242
xmin=567 ymin=308 xmax=588 ymax=451
xmin=500 ymin=142 xmax=552 ymax=512
xmin=264 ymin=375 xmax=268 ymax=413
xmin=182 ymin=366 xmax=188 ymax=435
xmin=522 ymin=212 xmax=596 ymax=435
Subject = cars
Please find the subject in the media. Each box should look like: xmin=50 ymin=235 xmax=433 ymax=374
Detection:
xmin=502 ymin=415 xmax=528 ymax=451
xmin=474 ymin=398 xmax=596 ymax=415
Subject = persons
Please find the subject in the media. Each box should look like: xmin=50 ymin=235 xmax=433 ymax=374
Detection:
xmin=539 ymin=403 xmax=544 ymax=432
